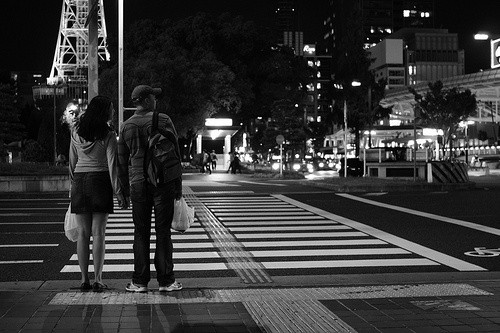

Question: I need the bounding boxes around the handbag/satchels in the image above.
[172,196,194,233]
[64,202,80,242]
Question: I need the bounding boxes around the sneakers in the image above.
[159,281,183,291]
[126,281,148,292]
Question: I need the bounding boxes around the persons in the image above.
[68,95,126,293]
[202,150,212,174]
[116,85,183,292]
[210,149,218,170]
[227,148,242,174]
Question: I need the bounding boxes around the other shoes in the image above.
[93,280,113,292]
[81,281,92,292]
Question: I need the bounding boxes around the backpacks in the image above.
[143,111,182,191]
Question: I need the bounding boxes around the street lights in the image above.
[473,30,491,42]
[351,81,362,87]
